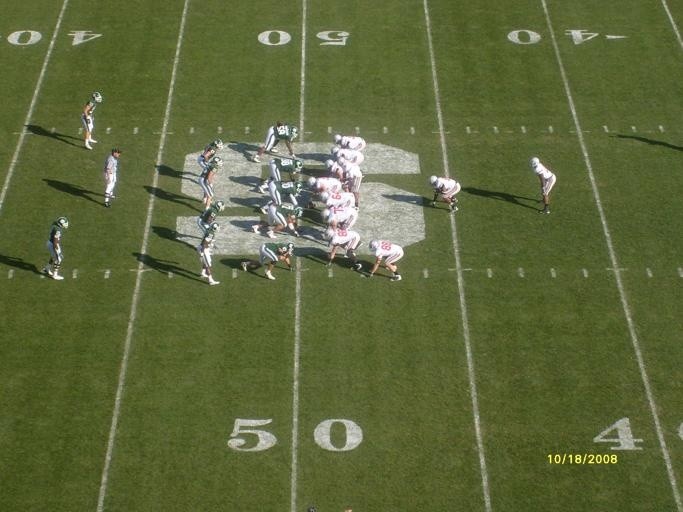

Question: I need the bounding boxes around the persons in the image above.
[368,240,404,281]
[241,121,367,281]
[104,148,123,208]
[530,157,556,214]
[81,91,103,150]
[45,216,70,282]
[430,175,461,212]
[197,137,225,287]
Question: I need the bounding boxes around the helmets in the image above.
[214,139,222,150]
[287,127,302,249]
[308,134,341,239]
[430,175,437,185]
[57,217,68,229]
[215,201,224,211]
[213,157,222,168]
[91,92,102,103]
[369,240,378,251]
[528,156,539,167]
[210,223,220,232]
